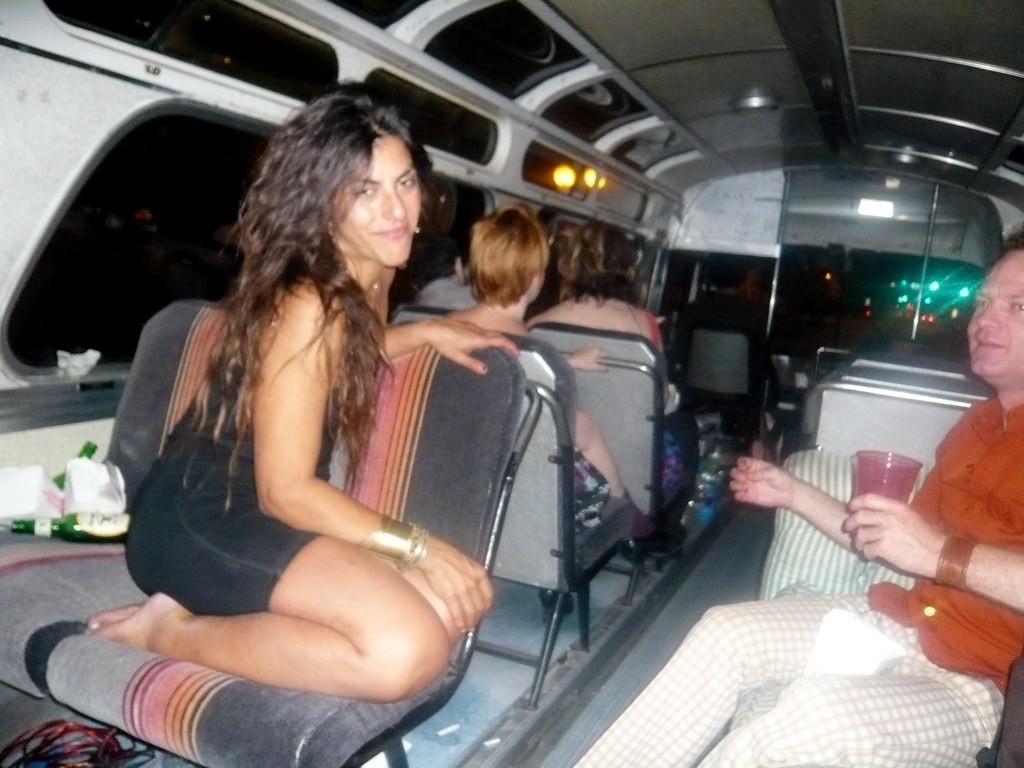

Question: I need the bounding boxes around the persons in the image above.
[714,264,783,451]
[448,202,654,549]
[85,89,520,703]
[413,254,478,309]
[526,216,684,503]
[572,227,1024,768]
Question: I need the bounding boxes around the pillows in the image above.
[758,449,935,601]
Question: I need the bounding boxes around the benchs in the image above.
[0,299,696,768]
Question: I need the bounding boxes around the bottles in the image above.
[13,512,129,543]
[54,441,96,490]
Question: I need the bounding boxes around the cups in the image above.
[855,450,924,506]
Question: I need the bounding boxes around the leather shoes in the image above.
[540,589,573,617]
[625,519,688,567]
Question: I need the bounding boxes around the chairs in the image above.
[685,326,769,451]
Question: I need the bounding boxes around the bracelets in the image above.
[936,536,976,590]
[370,517,429,568]
